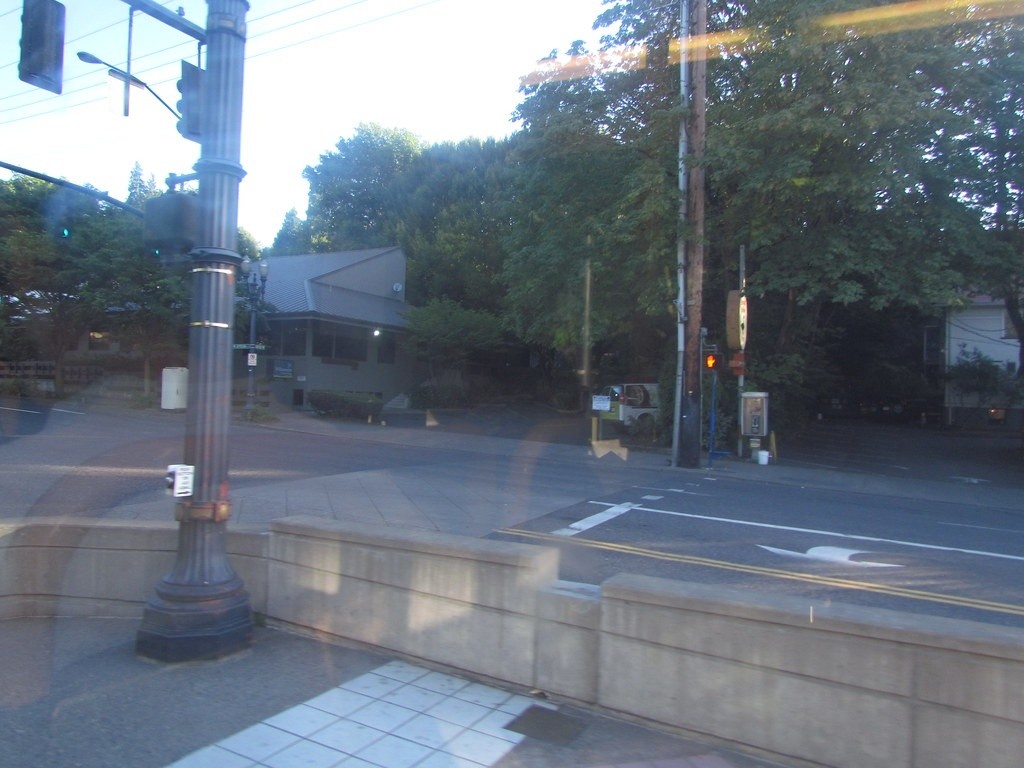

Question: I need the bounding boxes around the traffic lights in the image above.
[706,353,723,369]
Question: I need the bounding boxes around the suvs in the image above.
[598,381,665,437]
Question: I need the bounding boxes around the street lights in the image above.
[239,255,269,409]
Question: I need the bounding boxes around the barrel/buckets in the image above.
[758,450,769,465]
